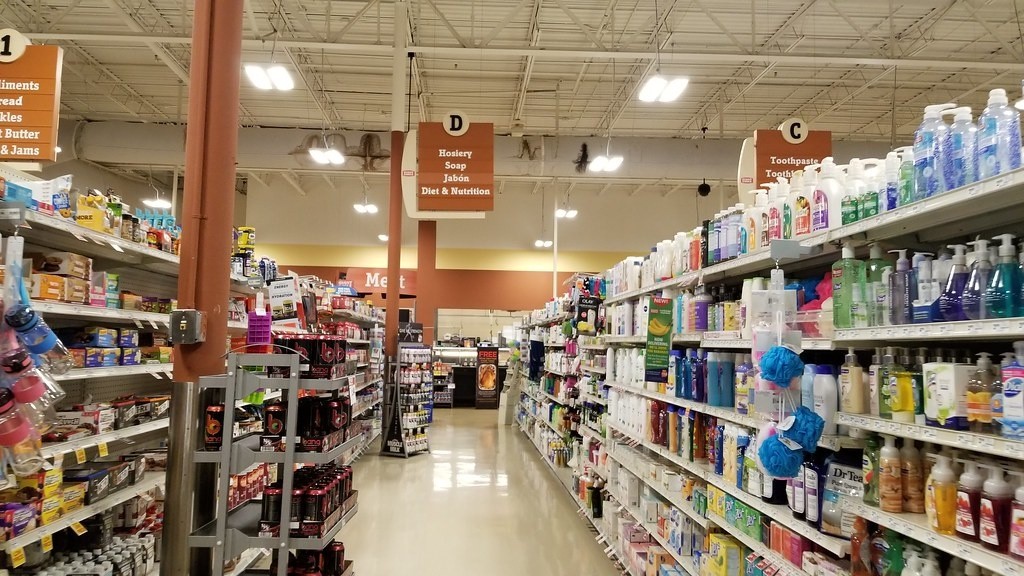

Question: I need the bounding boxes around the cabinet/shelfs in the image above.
[510,168,1024,575]
[0,202,390,576]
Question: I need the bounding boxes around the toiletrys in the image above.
[510,82,1024,576]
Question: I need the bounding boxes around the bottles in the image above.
[0,304,74,491]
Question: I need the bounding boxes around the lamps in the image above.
[243,35,293,92]
[352,186,378,214]
[307,123,345,166]
[639,40,688,103]
[556,195,577,220]
[588,138,623,172]
[534,229,553,248]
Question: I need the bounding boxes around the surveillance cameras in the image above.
[698,184,710,196]
